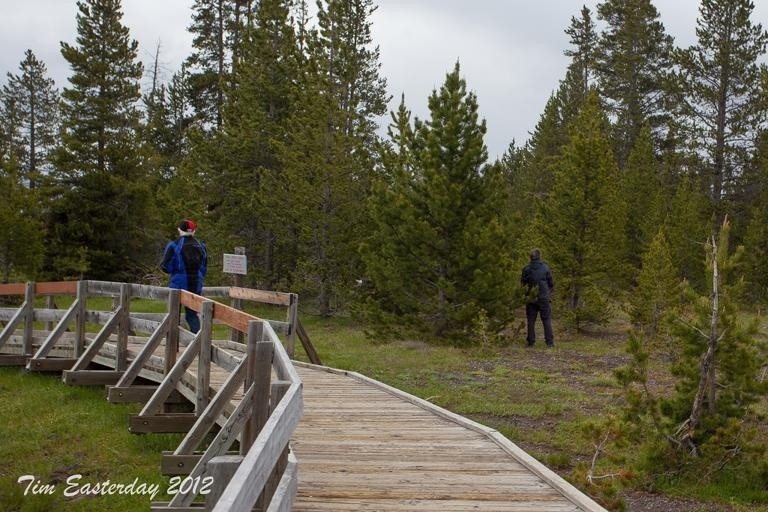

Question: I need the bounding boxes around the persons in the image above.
[158,219,208,335]
[520,248,556,348]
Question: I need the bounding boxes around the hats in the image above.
[180,220,195,232]
